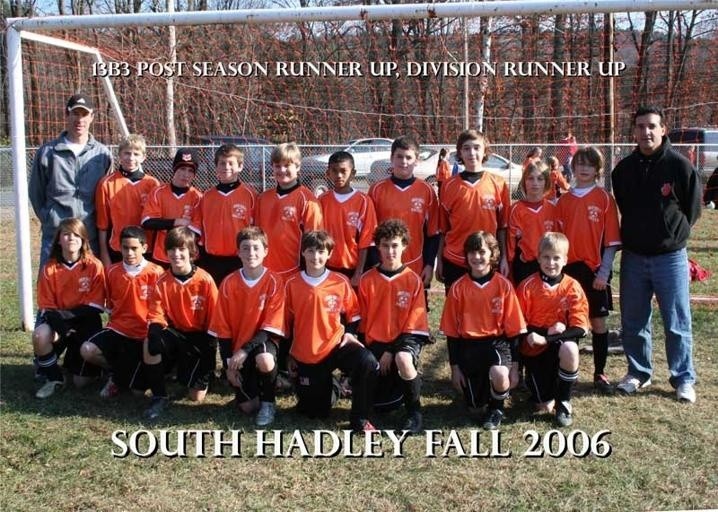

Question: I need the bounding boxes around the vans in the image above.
[666,127,718,183]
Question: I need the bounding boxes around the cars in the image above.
[366,144,523,197]
[300,138,396,177]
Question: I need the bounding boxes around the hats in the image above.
[66,94,94,114]
[173,148,199,178]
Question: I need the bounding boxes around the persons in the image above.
[437,148,450,199]
[79,226,164,398]
[208,227,290,426]
[33,219,105,400]
[523,147,543,169]
[286,231,382,431]
[141,149,204,270]
[518,232,589,426]
[684,145,695,164]
[567,136,577,181]
[704,155,718,207]
[506,161,557,284]
[28,95,113,283]
[543,156,571,197]
[256,142,323,277]
[435,129,511,295]
[613,106,702,404]
[614,132,636,161]
[558,146,623,393]
[439,231,528,429]
[202,144,258,287]
[358,220,430,428]
[556,129,573,183]
[318,151,378,286]
[368,136,439,311]
[143,227,218,401]
[96,134,160,267]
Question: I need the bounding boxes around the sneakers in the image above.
[533,398,555,418]
[572,378,580,394]
[351,413,384,436]
[676,382,696,403]
[555,400,573,427]
[143,394,168,423]
[402,411,423,434]
[616,374,652,394]
[98,367,107,384]
[219,367,230,386]
[208,371,219,384]
[99,377,120,400]
[274,372,293,395]
[593,374,615,396]
[339,370,352,399]
[36,370,72,399]
[288,361,306,388]
[255,402,276,426]
[189,388,207,402]
[165,369,179,384]
[483,409,505,430]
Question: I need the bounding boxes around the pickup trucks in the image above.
[142,135,330,198]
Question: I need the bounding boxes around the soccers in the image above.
[705,200,715,209]
